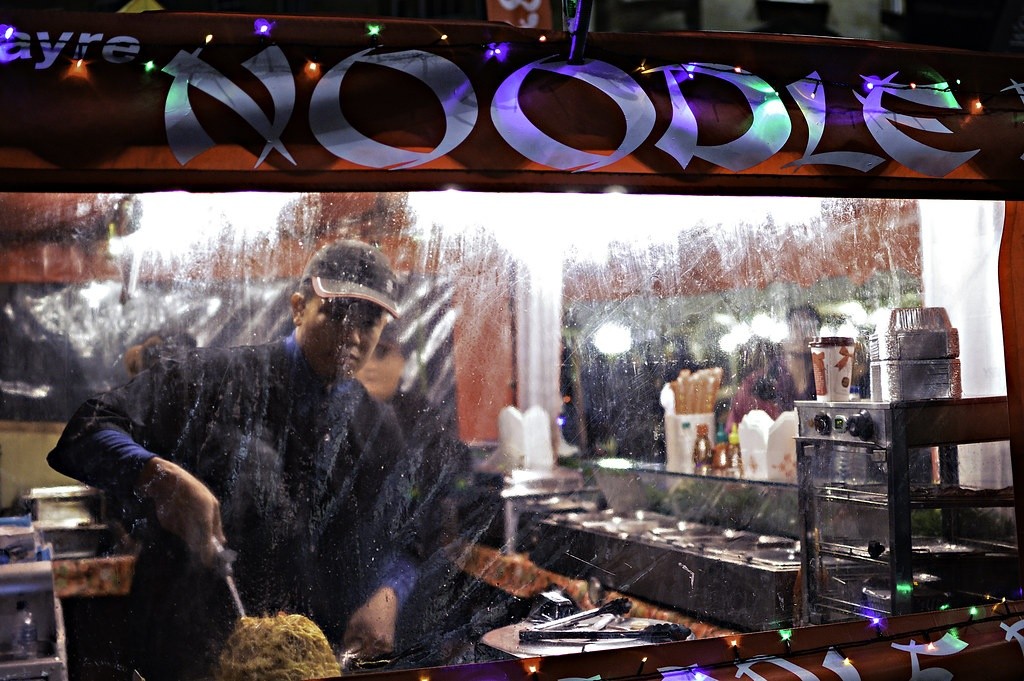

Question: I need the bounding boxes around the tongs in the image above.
[520,597,691,643]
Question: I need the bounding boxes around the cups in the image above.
[808,336,854,402]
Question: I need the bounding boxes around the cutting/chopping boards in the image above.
[481,616,694,657]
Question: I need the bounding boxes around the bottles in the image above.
[712,422,729,468]
[694,424,711,467]
[10,601,37,659]
[729,423,740,468]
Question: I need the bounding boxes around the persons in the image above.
[560,303,839,477]
[45,241,429,681]
[357,319,483,668]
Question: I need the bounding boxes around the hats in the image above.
[301,241,398,318]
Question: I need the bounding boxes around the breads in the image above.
[671,366,722,415]
